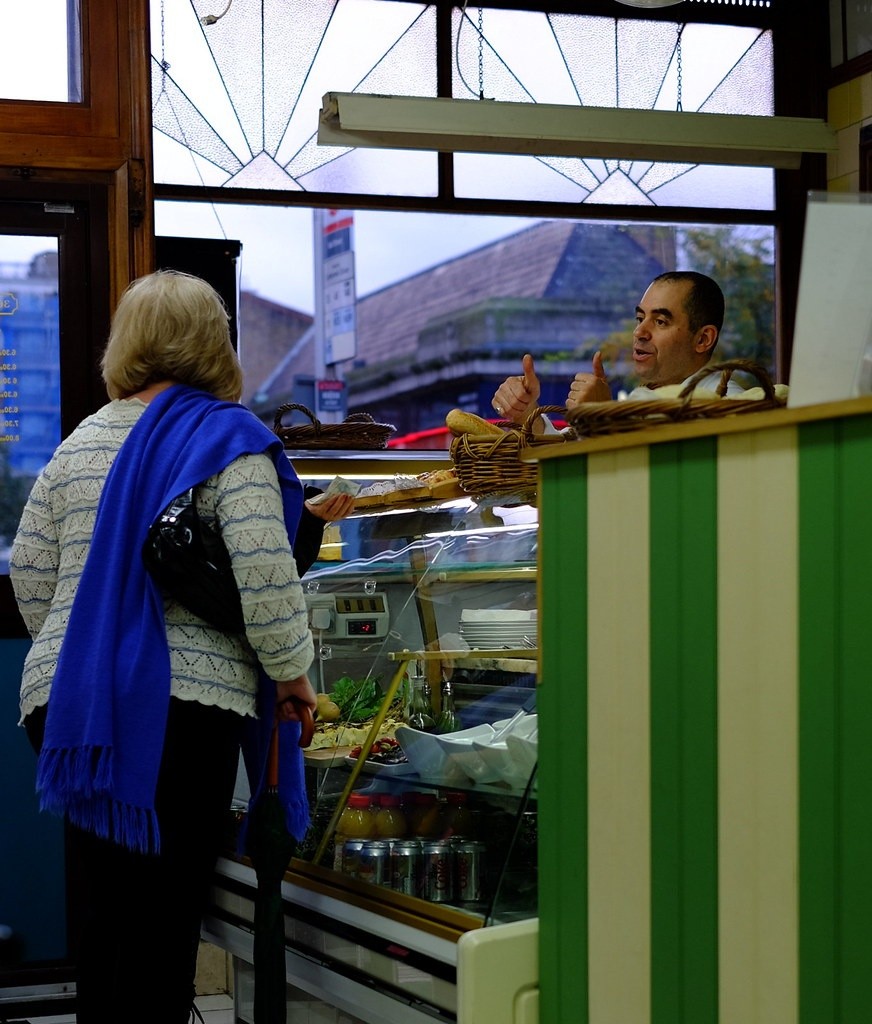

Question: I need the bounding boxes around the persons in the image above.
[491,271,745,426]
[10,271,354,1024]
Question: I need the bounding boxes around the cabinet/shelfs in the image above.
[200,485,539,1024]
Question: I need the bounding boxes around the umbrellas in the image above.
[246,697,315,1024]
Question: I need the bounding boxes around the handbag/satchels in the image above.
[144,482,247,640]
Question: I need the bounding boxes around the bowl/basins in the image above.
[394,715,538,791]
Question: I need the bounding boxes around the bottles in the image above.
[332,792,472,875]
[410,676,461,735]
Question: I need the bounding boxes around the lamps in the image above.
[317,0,839,171]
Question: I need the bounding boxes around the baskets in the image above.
[271,403,396,450]
[471,493,538,526]
[450,405,578,492]
[565,359,784,441]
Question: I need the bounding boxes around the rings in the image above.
[495,406,502,414]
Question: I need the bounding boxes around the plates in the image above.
[345,755,418,775]
[458,619,537,649]
